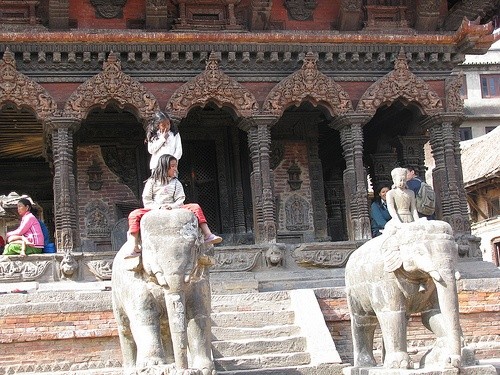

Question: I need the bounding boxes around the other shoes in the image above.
[204,234,222,245]
[124,251,141,259]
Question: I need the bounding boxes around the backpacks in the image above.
[413,178,435,215]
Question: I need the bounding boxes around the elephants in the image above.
[111,208,217,375]
[344,219,462,369]
